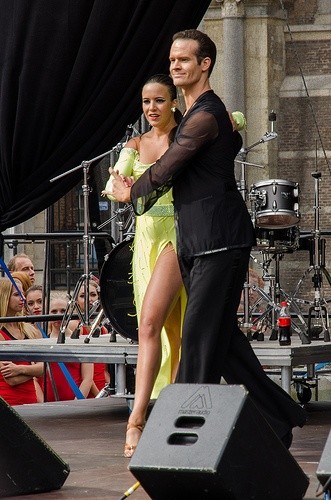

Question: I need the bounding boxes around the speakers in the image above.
[128,383,310,500]
[316,429,331,497]
[0,396,70,496]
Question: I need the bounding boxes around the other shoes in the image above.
[284,404,306,451]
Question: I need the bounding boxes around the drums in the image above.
[254,224,301,252]
[248,178,303,230]
[96,236,139,347]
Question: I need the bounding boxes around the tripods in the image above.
[49,141,122,344]
[239,178,331,345]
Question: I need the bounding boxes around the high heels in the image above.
[123,420,147,458]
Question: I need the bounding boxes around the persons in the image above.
[105,74,238,458]
[103,29,306,450]
[0,253,110,404]
[238,268,265,329]
